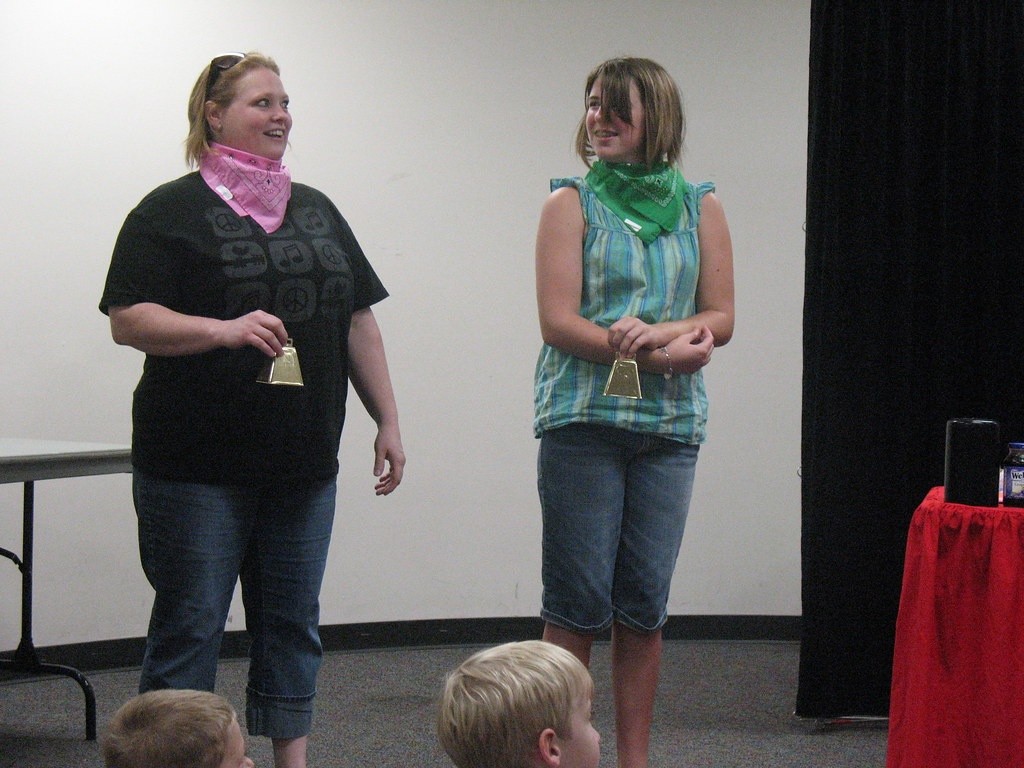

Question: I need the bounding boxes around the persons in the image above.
[98,52,407,768]
[103,689,255,768]
[436,640,602,768]
[531,56,736,768]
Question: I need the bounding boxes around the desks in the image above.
[0,437,131,741]
[887,485,1023,768]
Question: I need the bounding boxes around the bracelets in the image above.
[659,347,673,380]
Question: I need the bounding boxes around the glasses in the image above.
[205,52,245,104]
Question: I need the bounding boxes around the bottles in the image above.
[1001,442,1024,507]
